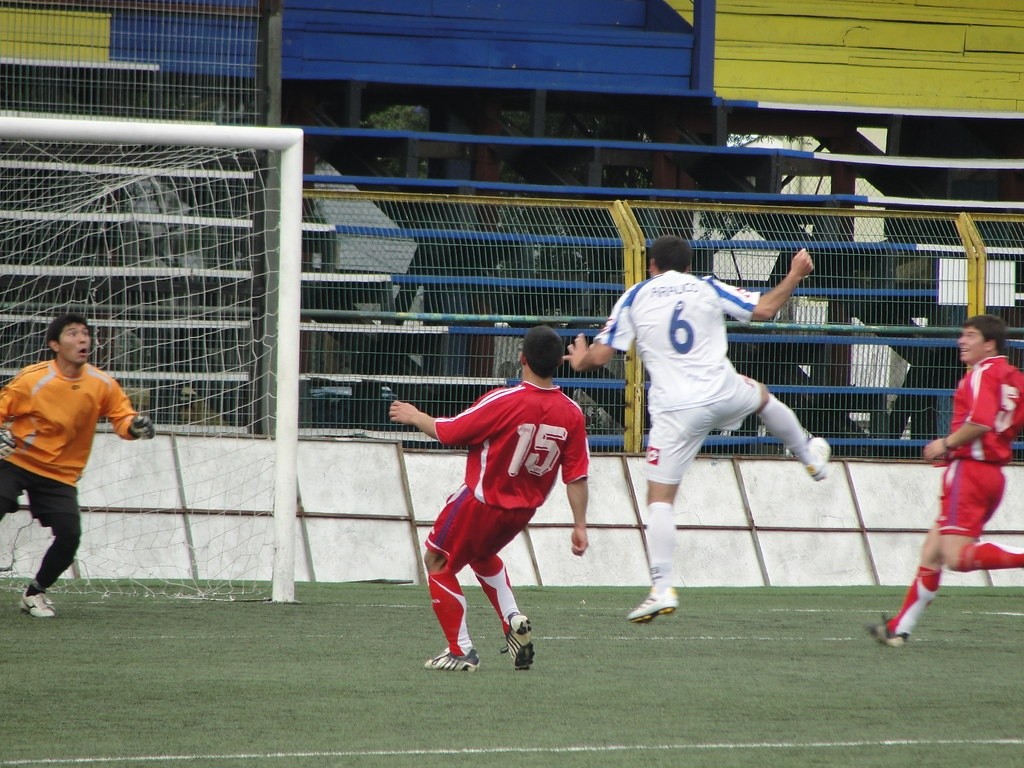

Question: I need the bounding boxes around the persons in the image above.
[387,327,590,672]
[862,314,1024,650]
[561,235,831,623]
[0,314,155,618]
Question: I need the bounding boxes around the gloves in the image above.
[0,428,15,460]
[130,416,155,439]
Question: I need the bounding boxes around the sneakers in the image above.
[867,621,909,647]
[805,437,831,479]
[425,648,480,672]
[627,587,678,623]
[21,588,56,617]
[505,612,534,670]
